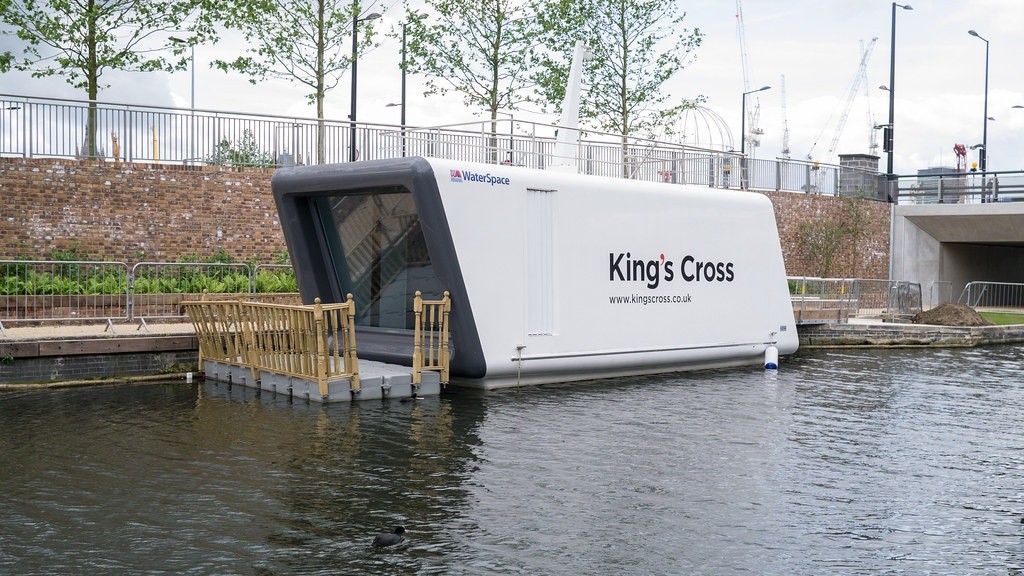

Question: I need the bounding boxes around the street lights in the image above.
[348,12,383,161]
[401,13,430,159]
[969,29,990,202]
[887,1,914,174]
[742,86,772,179]
[169,36,195,168]
